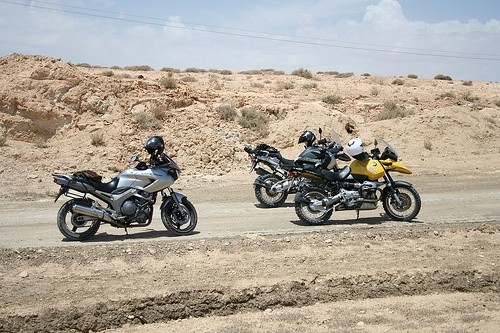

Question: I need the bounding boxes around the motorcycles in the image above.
[244,128,351,207]
[51,135,197,238]
[293,124,421,225]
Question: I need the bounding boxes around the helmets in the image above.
[146,135,164,154]
[298,130,316,148]
[346,138,363,156]
